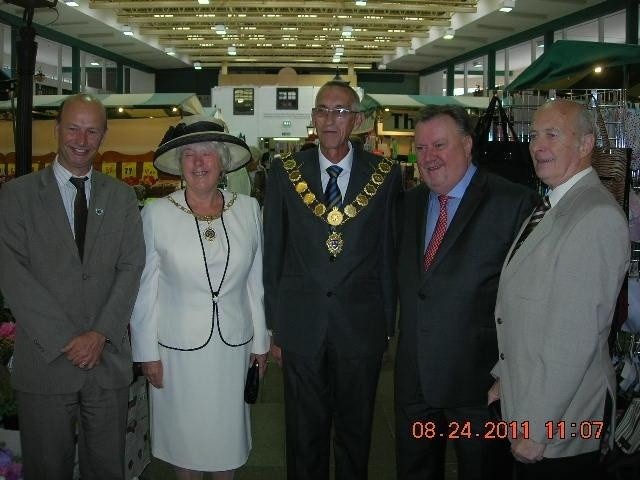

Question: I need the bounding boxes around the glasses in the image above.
[313,106,353,119]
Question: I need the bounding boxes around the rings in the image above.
[81,361,88,367]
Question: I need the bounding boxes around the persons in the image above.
[263,81,400,480]
[487,98,630,480]
[128,115,273,480]
[1,93,147,479]
[226,146,267,207]
[394,104,537,480]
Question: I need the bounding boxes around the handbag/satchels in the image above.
[244,359,261,404]
[591,147,631,211]
[479,140,537,190]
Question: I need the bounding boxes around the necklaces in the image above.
[280,151,394,259]
[164,190,238,243]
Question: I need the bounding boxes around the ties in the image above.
[326,165,344,214]
[68,174,88,263]
[507,194,551,264]
[423,195,449,269]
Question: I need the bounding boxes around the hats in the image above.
[152,116,252,177]
